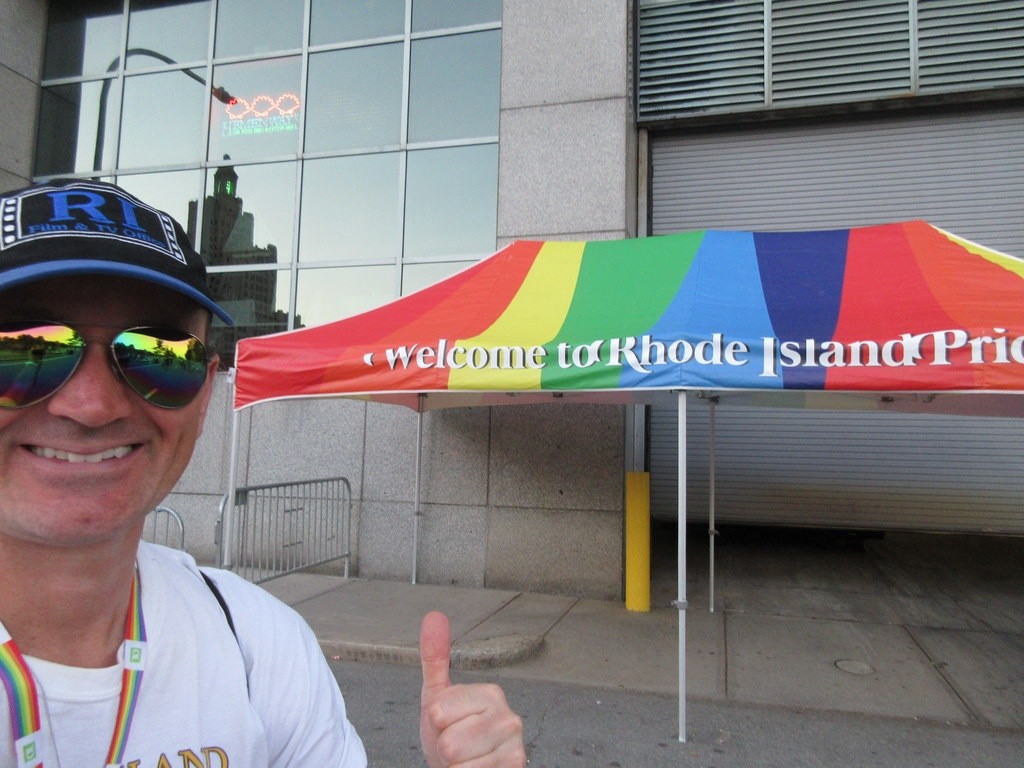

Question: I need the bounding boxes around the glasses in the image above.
[0,318,216,412]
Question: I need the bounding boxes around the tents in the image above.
[220,218,1024,745]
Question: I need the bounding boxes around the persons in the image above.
[0,179,527,768]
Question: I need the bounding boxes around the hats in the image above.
[0,178,237,329]
